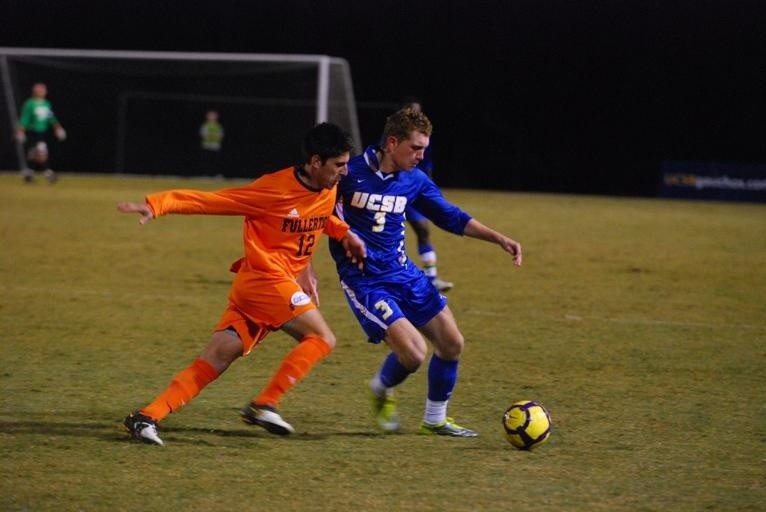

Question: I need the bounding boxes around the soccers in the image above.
[501,400,552,449]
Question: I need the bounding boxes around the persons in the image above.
[332,102,522,439]
[15,82,66,184]
[200,111,225,180]
[383,103,456,293]
[118,125,367,445]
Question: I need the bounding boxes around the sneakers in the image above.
[124,409,163,445]
[366,380,399,433]
[418,416,478,437]
[431,278,454,292]
[240,402,294,435]
